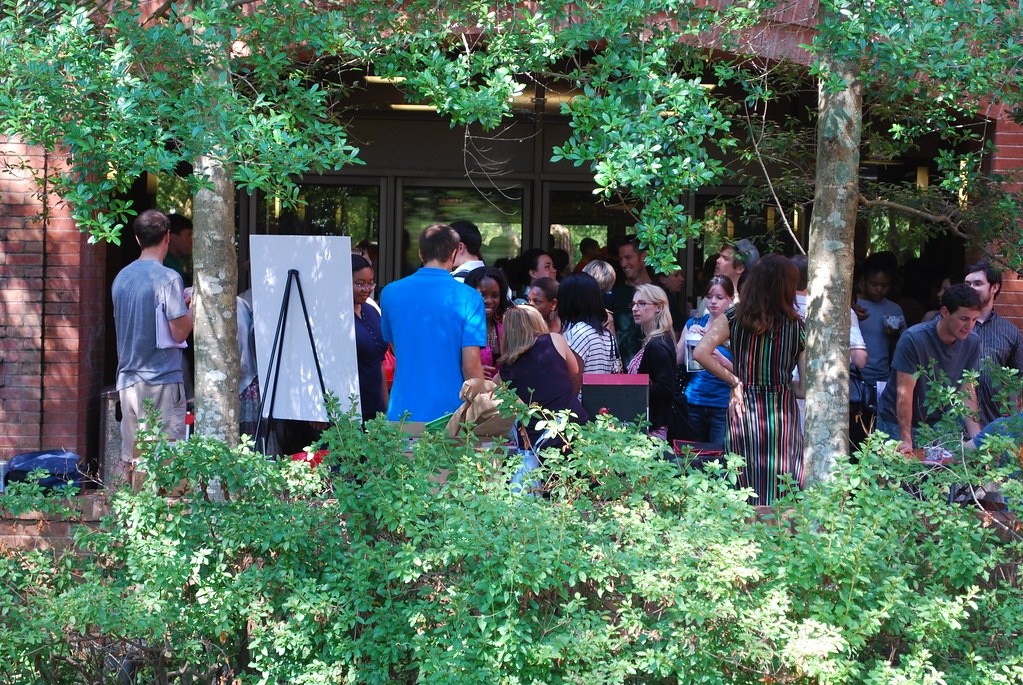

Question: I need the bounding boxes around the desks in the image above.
[907,446,982,511]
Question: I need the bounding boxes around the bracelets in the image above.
[729,376,741,390]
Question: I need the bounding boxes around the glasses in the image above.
[631,300,658,308]
[625,235,639,242]
[353,281,377,290]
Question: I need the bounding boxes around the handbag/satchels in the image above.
[849,362,877,433]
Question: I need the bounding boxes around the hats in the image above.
[735,238,760,276]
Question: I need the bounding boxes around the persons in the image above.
[573,238,601,271]
[381,226,487,423]
[627,283,679,445]
[527,277,565,335]
[693,252,808,507]
[110,208,192,488]
[162,214,194,404]
[698,238,760,313]
[547,248,571,279]
[448,219,485,283]
[235,295,280,459]
[464,266,512,381]
[555,271,620,404]
[678,274,736,445]
[582,260,617,340]
[487,304,600,498]
[506,248,557,306]
[310,252,389,451]
[612,235,686,374]
[789,248,1023,512]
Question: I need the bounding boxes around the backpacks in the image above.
[3,449,83,497]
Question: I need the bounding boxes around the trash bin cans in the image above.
[97,388,131,494]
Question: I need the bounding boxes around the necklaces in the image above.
[487,321,498,352]
